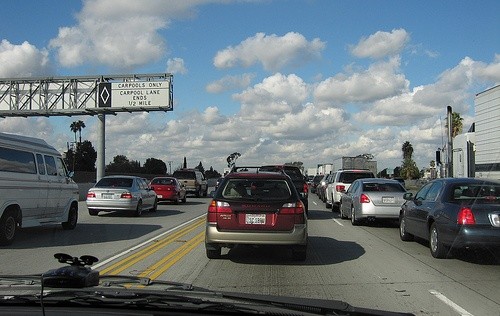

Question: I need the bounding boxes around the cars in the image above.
[338,178,409,226]
[399,177,500,259]
[205,166,309,260]
[86,176,159,217]
[305,173,337,202]
[148,177,187,204]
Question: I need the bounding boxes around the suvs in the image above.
[324,169,381,213]
[173,168,208,198]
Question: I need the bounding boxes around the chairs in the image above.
[475,186,491,197]
[462,189,472,196]
[230,184,247,196]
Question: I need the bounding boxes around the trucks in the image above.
[0,133,79,247]
